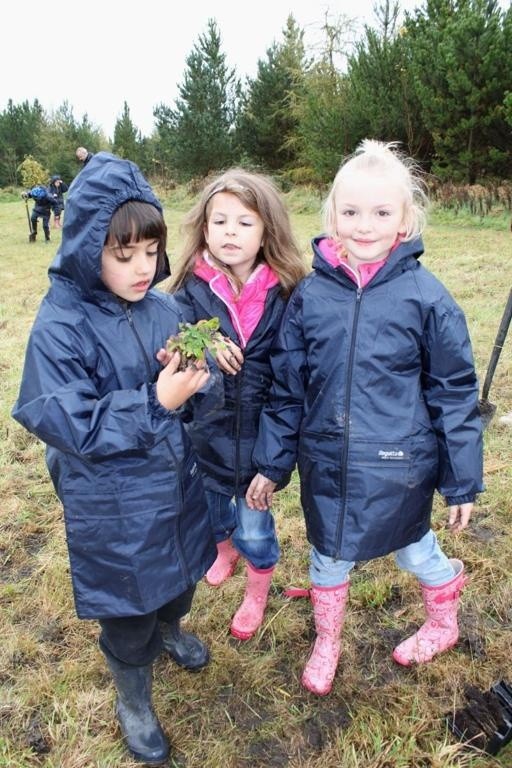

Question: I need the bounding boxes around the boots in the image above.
[30,220,39,235]
[228,557,276,641]
[392,556,466,667]
[97,630,171,765]
[154,615,211,674]
[44,230,52,244]
[204,535,243,587]
[281,580,352,696]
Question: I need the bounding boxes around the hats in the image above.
[29,186,46,200]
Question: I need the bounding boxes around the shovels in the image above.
[478,287,512,427]
[23,196,37,242]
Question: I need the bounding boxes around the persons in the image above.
[22,187,61,241]
[76,147,94,168]
[11,150,224,767]
[164,167,311,641]
[246,138,486,695]
[47,176,68,230]
